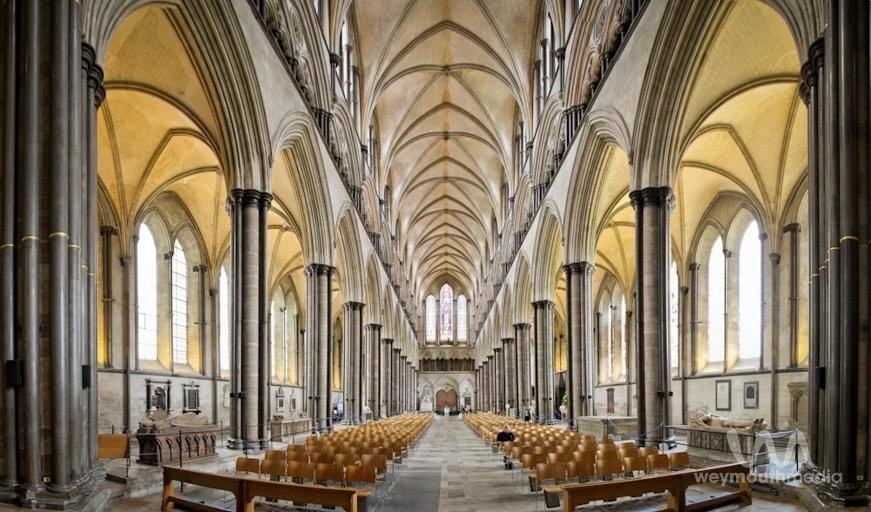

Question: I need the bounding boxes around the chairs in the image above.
[462,410,705,512]
[223,411,435,512]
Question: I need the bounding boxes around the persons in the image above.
[685,407,768,434]
[135,410,211,429]
[559,401,568,422]
[442,403,473,423]
[497,424,515,471]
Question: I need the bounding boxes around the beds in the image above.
[134,423,234,467]
[688,410,769,455]
